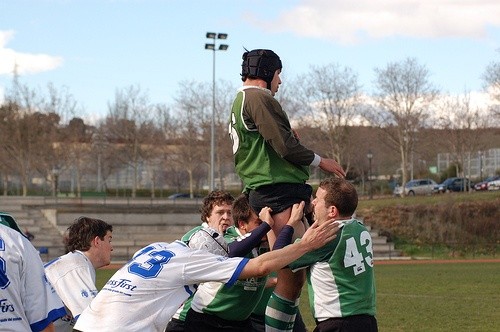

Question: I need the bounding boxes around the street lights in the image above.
[204,32,228,193]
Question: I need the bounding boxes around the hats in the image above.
[240,49,282,83]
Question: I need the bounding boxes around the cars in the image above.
[432,176,475,193]
[394,178,438,196]
[474,175,500,191]
[168,193,201,200]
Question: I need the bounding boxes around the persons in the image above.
[44,217,114,321]
[165,190,235,332]
[272,177,378,332]
[184,195,275,332]
[229,50,347,332]
[73,219,344,332]
[0,215,67,332]
